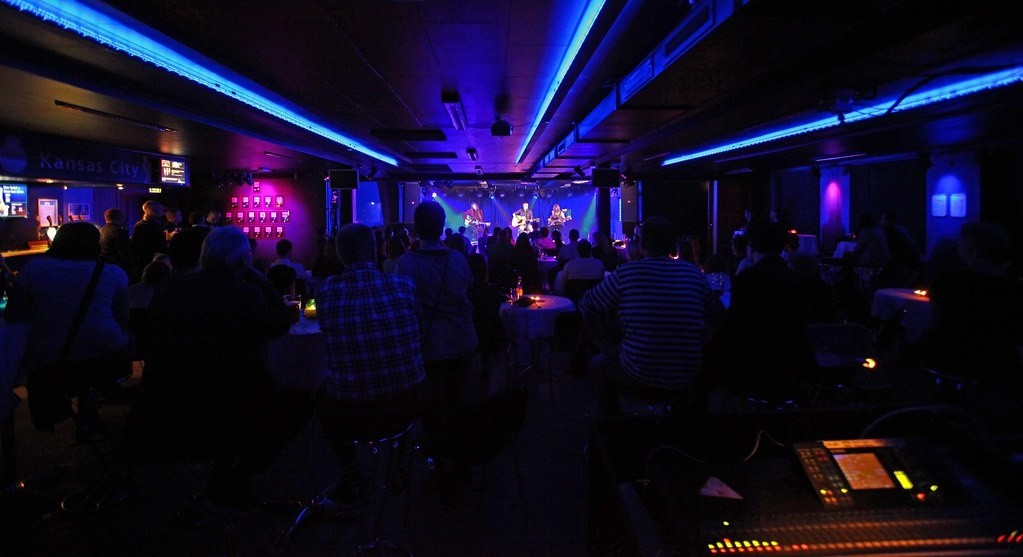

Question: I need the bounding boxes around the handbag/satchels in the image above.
[27,342,81,433]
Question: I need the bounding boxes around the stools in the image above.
[203,384,315,512]
[34,364,136,531]
[318,385,417,529]
[126,384,217,521]
[419,388,527,512]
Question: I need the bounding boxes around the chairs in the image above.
[870,309,908,370]
[564,278,602,304]
[808,323,876,374]
[536,312,581,399]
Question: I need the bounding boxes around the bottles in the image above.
[515,276,523,298]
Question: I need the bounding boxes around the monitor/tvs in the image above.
[158,156,188,187]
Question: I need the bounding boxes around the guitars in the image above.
[511,214,541,228]
[464,217,492,228]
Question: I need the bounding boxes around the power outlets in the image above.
[466,148,479,162]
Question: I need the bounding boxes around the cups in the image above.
[284,294,302,324]
[510,289,519,305]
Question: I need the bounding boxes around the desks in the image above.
[831,241,857,259]
[869,289,937,358]
[781,234,819,259]
[262,310,330,397]
[535,256,558,290]
[498,294,576,385]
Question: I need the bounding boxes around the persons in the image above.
[0,147,1023,513]
[462,202,483,236]
[548,204,566,231]
[512,202,534,232]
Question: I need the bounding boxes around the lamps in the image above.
[620,172,636,187]
[574,167,585,178]
[244,177,254,186]
[365,169,378,180]
[440,92,468,130]
[475,167,483,175]
